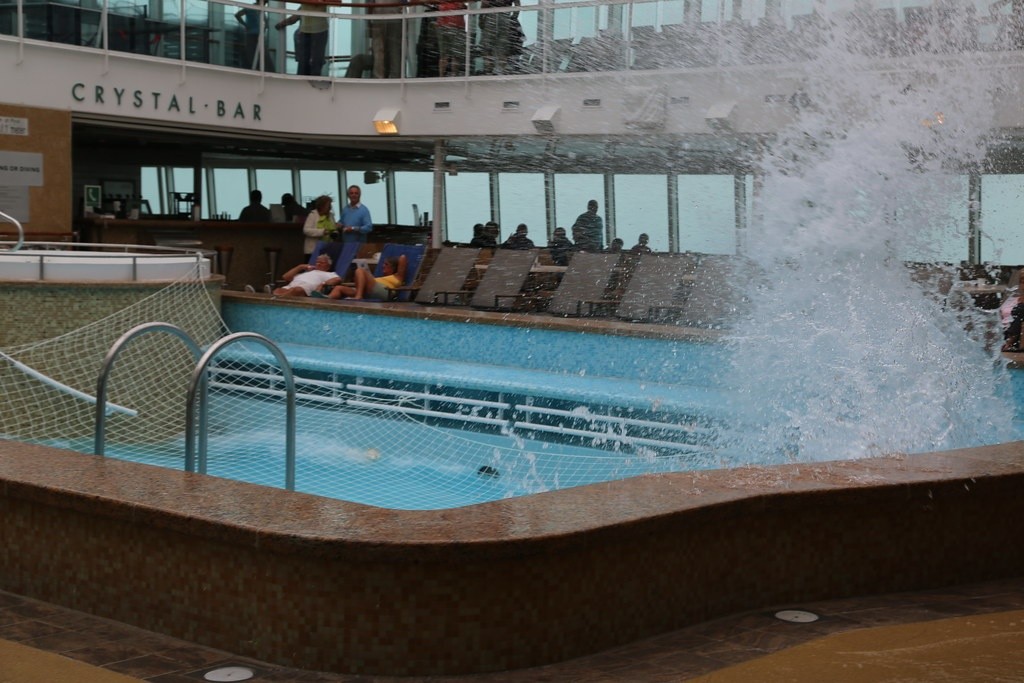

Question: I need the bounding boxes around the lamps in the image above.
[373,108,403,135]
[705,102,738,130]
[529,108,562,134]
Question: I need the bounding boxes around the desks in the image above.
[950,286,1006,311]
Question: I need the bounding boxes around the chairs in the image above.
[515,5,975,74]
[273,241,756,328]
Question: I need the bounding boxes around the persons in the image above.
[233,0,277,73]
[310,253,407,301]
[274,0,330,76]
[569,199,605,249]
[470,221,499,245]
[605,238,624,253]
[280,192,308,221]
[334,184,374,234]
[244,254,344,296]
[630,233,652,252]
[303,194,338,250]
[413,0,524,75]
[502,223,535,247]
[545,227,574,267]
[238,190,272,223]
[365,0,404,77]
